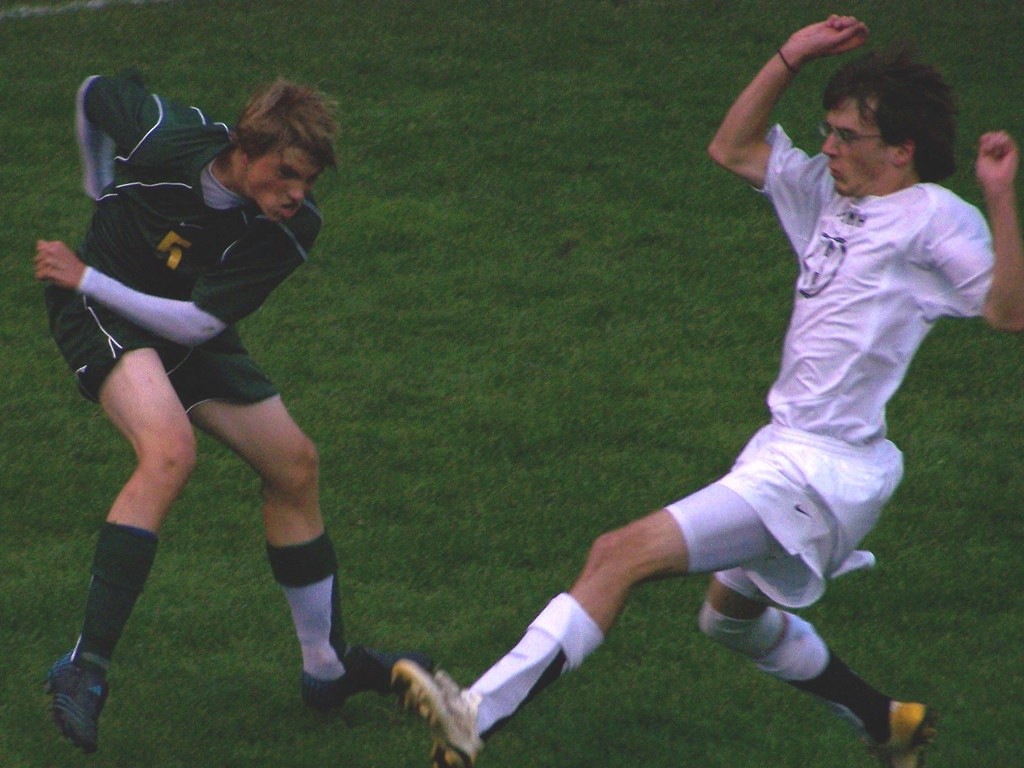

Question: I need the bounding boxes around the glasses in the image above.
[818,119,884,144]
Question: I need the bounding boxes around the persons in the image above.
[387,13,1024,768]
[28,72,435,757]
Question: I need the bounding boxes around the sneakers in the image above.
[301,644,434,713]
[390,655,486,768]
[862,699,939,768]
[45,649,109,754]
